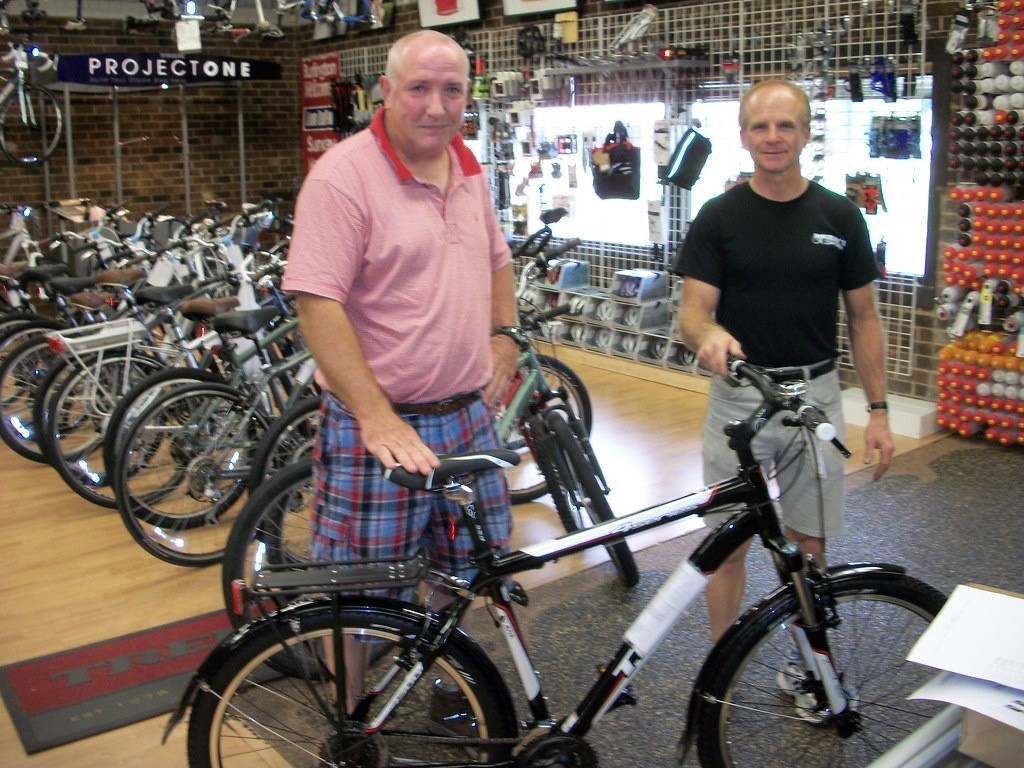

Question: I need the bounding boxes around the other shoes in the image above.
[430,684,484,745]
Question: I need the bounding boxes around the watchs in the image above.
[865,402,887,411]
[489,325,530,353]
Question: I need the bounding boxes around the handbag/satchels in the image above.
[588,129,641,200]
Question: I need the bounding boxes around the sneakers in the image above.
[777,659,830,724]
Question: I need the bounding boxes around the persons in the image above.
[279,30,521,762]
[675,78,895,723]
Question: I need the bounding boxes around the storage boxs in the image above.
[517,257,715,377]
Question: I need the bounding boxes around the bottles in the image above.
[936,0,1024,447]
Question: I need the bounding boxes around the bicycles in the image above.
[0,8,63,170]
[0,192,640,589]
[162,350,951,768]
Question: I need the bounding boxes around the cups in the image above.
[844,67,864,104]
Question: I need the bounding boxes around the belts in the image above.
[390,389,480,416]
[728,360,834,384]
